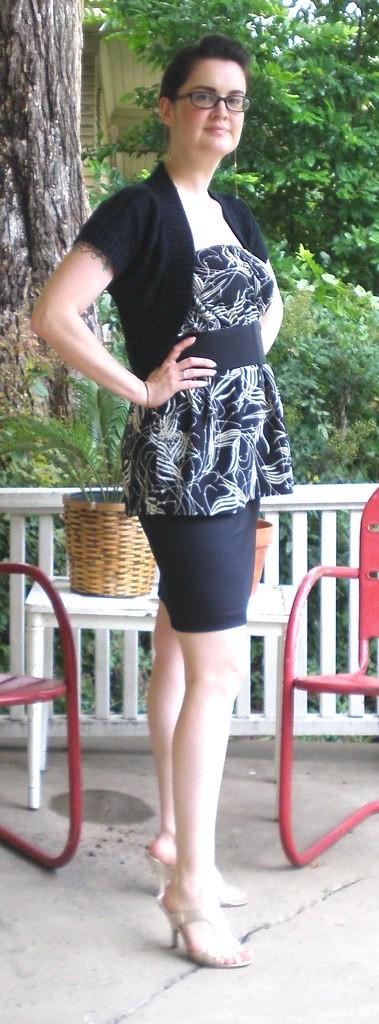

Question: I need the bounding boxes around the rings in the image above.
[183,370,188,379]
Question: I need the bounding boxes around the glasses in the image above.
[172,90,253,113]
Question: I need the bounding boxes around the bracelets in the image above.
[144,382,149,405]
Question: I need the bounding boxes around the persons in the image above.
[29,33,295,969]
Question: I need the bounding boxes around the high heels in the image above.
[146,831,248,909]
[156,890,253,968]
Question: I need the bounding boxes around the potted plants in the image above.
[0,338,157,598]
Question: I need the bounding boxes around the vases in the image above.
[248,519,273,596]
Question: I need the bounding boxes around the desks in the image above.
[23,574,299,821]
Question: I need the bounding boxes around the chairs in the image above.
[279,486,379,865]
[0,562,83,871]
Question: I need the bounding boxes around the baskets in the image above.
[62,490,155,599]
